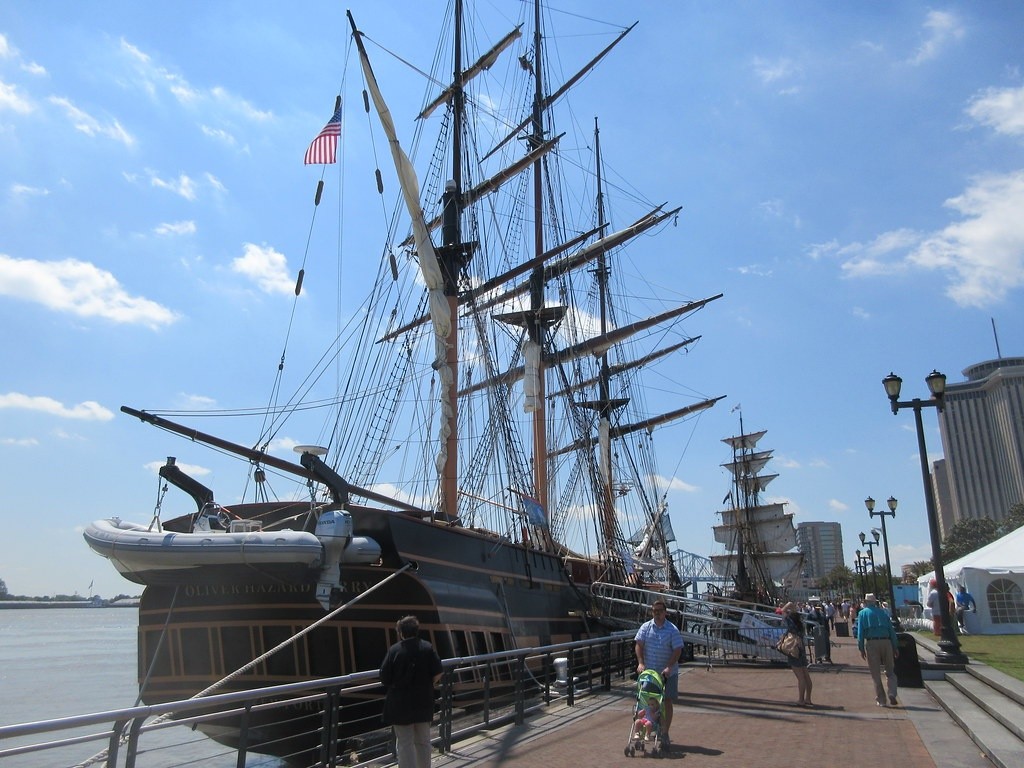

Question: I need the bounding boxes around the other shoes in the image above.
[645,734,649,741]
[825,657,831,661]
[876,701,886,707]
[817,658,822,664]
[634,732,640,740]
[661,735,669,746]
[888,693,898,705]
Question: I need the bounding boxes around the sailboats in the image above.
[84,2,730,764]
[690,409,809,641]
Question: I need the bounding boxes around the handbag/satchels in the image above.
[776,625,802,659]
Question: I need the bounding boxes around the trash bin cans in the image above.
[887,632,924,690]
[834,621,849,637]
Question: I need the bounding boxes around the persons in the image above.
[631,697,663,742]
[903,599,923,618]
[303,96,343,167]
[767,592,900,665]
[784,612,814,707]
[378,614,444,768]
[857,593,900,707]
[633,600,685,753]
[926,578,976,636]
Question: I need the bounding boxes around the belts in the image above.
[867,637,890,641]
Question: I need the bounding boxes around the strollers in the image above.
[624,670,672,760]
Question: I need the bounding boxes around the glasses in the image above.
[654,609,665,612]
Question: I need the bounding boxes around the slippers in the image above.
[790,701,813,707]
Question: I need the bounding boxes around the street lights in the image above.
[865,494,905,633]
[859,531,880,608]
[854,559,867,599]
[856,548,872,593]
[882,369,970,665]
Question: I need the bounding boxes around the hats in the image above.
[864,593,876,601]
[814,603,821,608]
